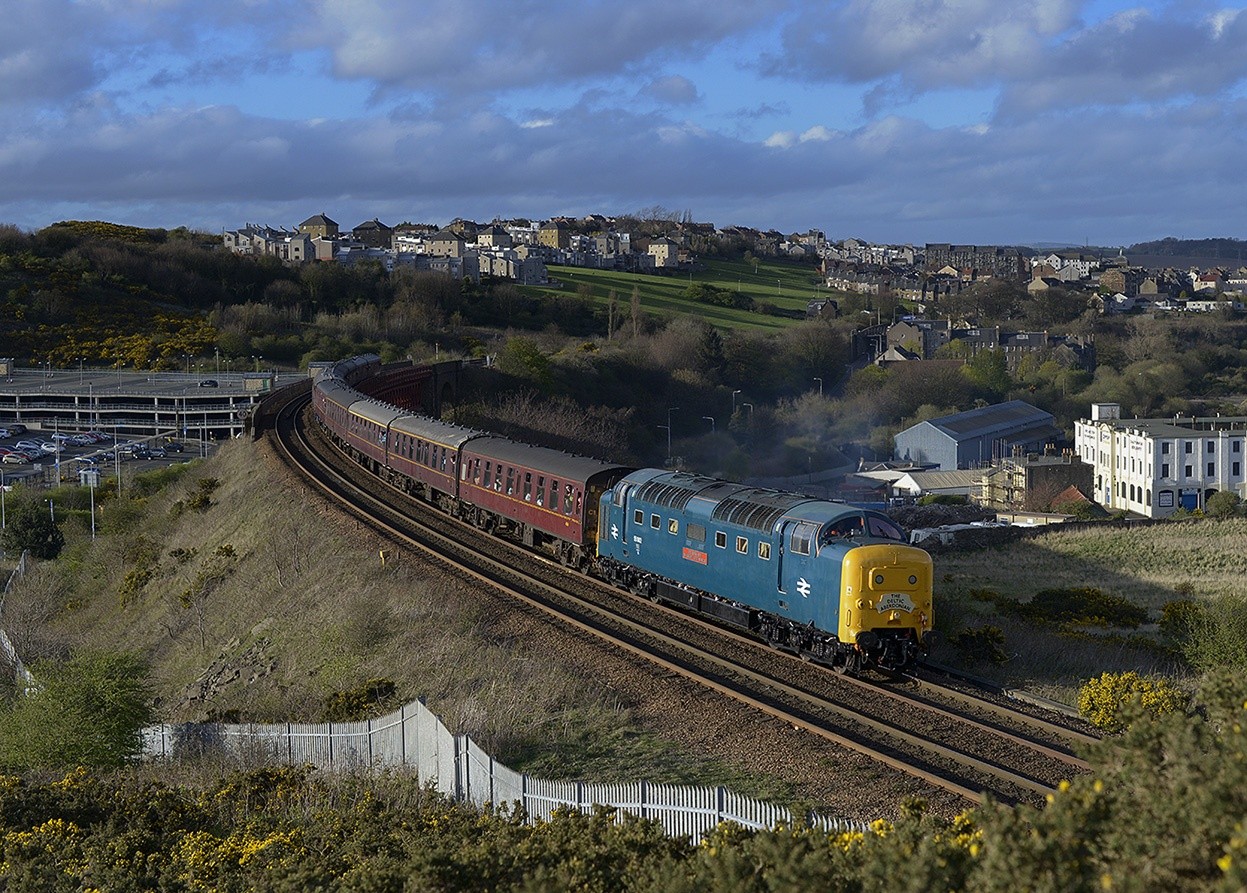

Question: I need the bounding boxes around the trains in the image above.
[311,350,938,678]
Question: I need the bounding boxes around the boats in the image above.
[1,422,191,493]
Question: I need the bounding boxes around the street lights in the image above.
[3,346,267,392]
